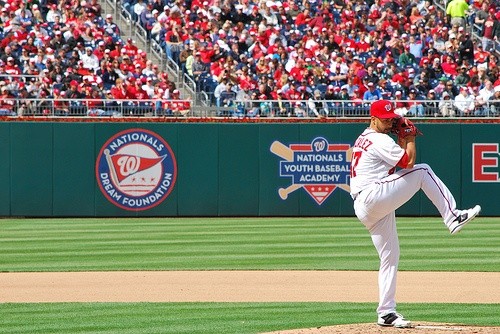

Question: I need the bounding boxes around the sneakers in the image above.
[449,204,481,234]
[378,313,414,329]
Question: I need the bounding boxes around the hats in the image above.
[370,101,400,119]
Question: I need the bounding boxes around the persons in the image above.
[0,0,192,117]
[350,101,481,326]
[113,0,500,117]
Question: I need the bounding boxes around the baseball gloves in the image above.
[390,115,424,139]
